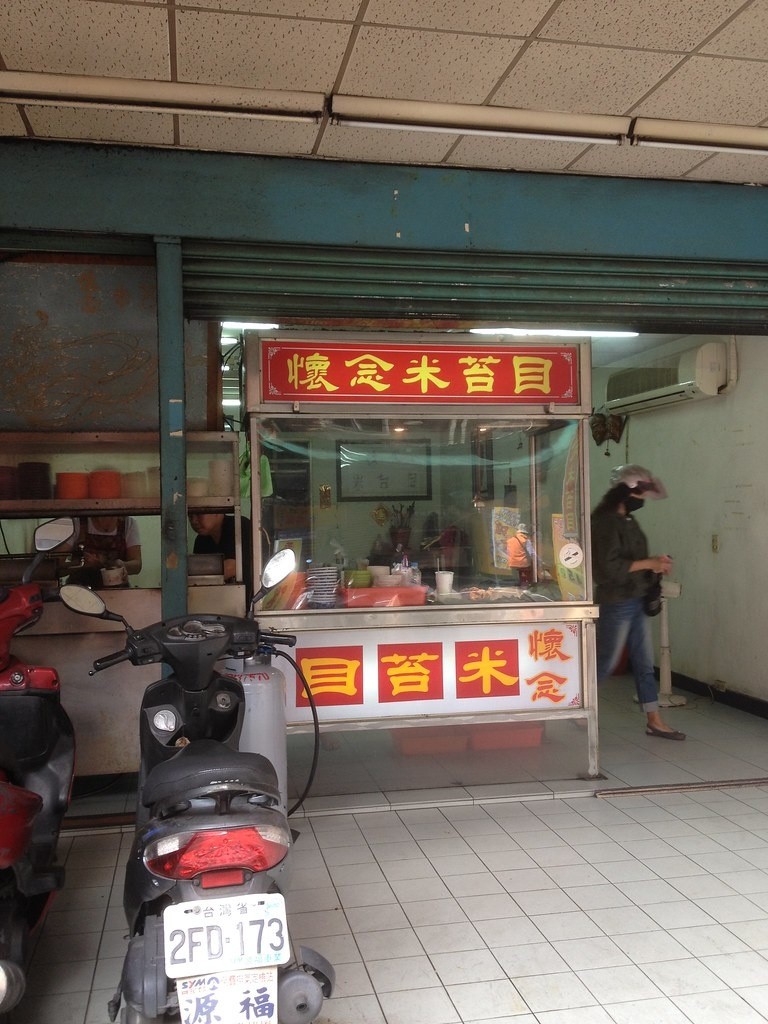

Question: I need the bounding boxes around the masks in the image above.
[623,496,644,513]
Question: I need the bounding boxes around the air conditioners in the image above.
[605,334,737,417]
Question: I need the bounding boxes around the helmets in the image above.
[609,463,668,500]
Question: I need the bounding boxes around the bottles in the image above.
[401,555,409,572]
[410,562,421,585]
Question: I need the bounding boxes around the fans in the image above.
[633,573,688,707]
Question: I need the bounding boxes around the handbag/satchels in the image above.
[644,570,663,616]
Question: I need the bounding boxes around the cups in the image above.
[435,571,454,603]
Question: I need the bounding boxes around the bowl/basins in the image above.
[146,467,160,497]
[56,473,88,499]
[0,466,18,499]
[208,461,232,496]
[89,471,120,499]
[123,472,146,498]
[344,565,401,588]
[18,462,50,498]
[101,567,124,585]
[307,567,338,608]
[188,479,209,497]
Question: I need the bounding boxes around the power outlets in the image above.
[713,680,726,692]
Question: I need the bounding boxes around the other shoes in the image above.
[646,723,685,740]
[571,719,600,732]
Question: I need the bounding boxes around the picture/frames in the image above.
[335,438,433,502]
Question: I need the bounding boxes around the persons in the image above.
[187,511,272,585]
[53,516,142,588]
[506,523,536,588]
[591,464,688,743]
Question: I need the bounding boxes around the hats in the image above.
[517,524,528,533]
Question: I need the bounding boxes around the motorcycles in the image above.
[0,514,80,1024]
[57,549,335,1024]
